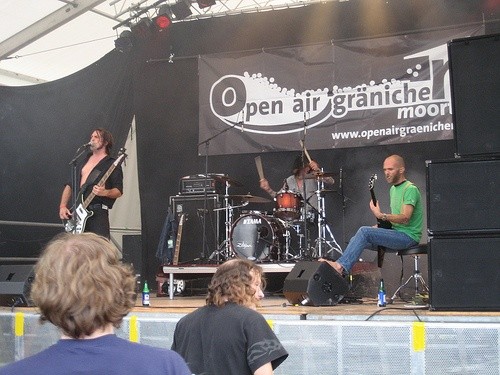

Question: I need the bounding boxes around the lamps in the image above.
[114,0,193,54]
[196,0,216,9]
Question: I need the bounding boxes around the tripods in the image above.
[279,177,344,262]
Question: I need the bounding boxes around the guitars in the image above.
[368,173,392,268]
[62,151,127,235]
[173,213,190,265]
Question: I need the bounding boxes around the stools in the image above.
[397,245,429,309]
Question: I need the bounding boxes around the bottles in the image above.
[376,279,386,307]
[141,280,149,307]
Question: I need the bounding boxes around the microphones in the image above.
[304,112,306,135]
[241,110,244,132]
[197,209,208,214]
[83,142,92,147]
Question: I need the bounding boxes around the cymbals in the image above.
[299,171,338,180]
[219,194,272,204]
[210,175,243,188]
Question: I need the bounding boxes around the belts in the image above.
[86,203,109,211]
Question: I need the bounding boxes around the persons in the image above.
[260,154,335,211]
[318,155,423,276]
[60,128,123,240]
[172,259,289,375]
[0,232,192,375]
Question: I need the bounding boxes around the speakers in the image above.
[283,261,350,308]
[425,32,500,313]
[0,265,36,307]
[169,195,227,264]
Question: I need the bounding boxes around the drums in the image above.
[275,191,302,220]
[230,214,291,262]
